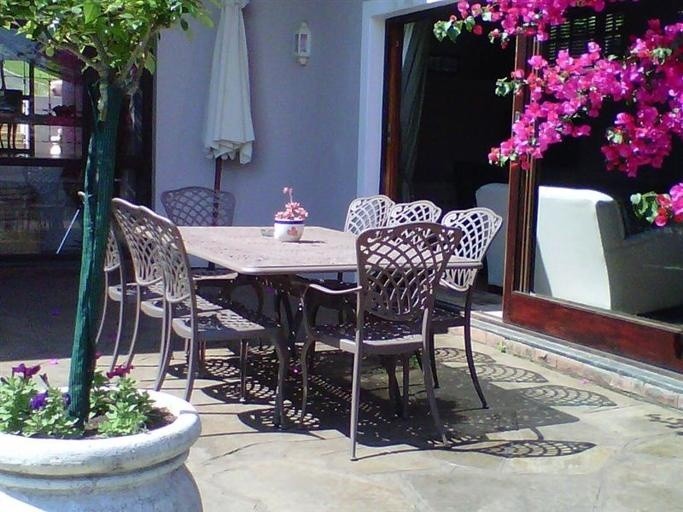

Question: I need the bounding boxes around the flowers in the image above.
[0,352,169,441]
[272,186,309,221]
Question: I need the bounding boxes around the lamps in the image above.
[290,19,312,68]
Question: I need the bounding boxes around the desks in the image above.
[173,223,485,437]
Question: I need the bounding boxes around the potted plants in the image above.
[0,0,209,511]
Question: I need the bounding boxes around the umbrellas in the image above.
[182,1,256,284]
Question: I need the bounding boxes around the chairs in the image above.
[111,197,220,383]
[158,185,239,306]
[135,205,265,403]
[316,199,444,355]
[294,222,464,461]
[296,193,395,351]
[375,201,502,412]
[76,191,161,372]
[0,160,73,236]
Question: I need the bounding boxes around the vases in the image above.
[274,219,304,242]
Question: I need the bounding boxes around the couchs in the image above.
[474,180,683,317]
